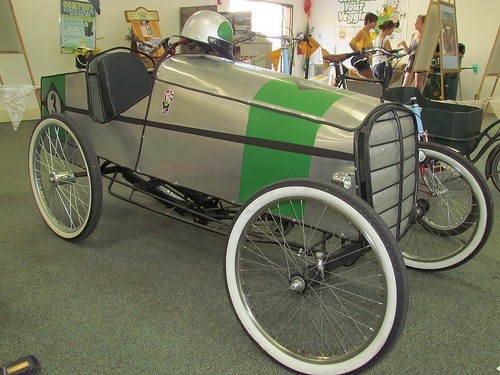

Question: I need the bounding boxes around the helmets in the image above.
[173,10,236,61]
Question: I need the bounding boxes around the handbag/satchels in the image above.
[378,66,393,84]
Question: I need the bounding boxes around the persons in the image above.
[373,18,396,85]
[349,13,379,78]
[398,15,426,95]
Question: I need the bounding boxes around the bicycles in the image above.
[322,48,410,99]
